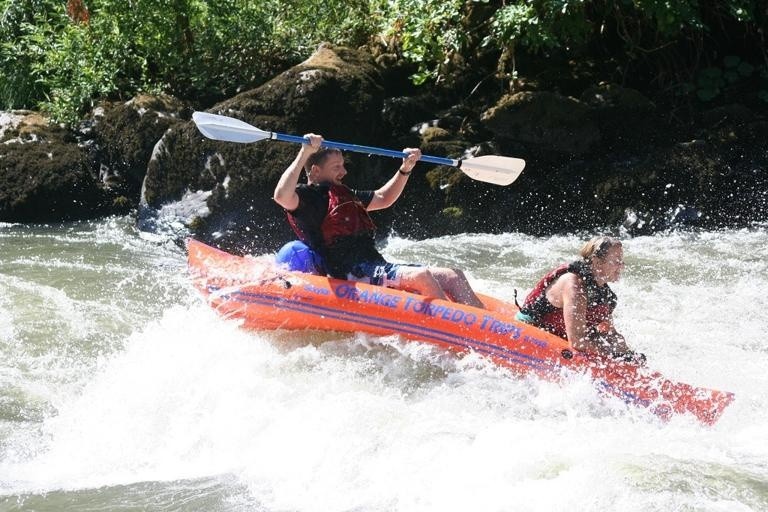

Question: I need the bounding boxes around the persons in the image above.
[272,130,488,310]
[515,234,647,369]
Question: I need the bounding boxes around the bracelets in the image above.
[398,168,413,176]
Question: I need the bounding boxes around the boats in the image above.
[180,235,735,430]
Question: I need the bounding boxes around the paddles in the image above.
[192,110,526,186]
